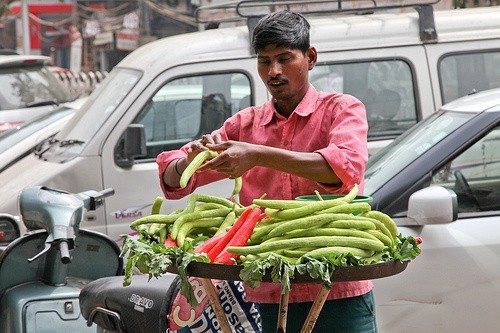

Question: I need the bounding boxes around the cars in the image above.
[0,55,250,174]
[364,88,500,333]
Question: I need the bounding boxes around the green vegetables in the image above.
[119,232,421,307]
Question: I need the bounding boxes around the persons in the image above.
[156,11,379,332]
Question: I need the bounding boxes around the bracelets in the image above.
[175,157,184,178]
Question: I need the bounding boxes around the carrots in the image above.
[194,207,267,266]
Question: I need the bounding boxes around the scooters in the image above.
[0,185,180,332]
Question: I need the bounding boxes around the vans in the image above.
[0,5,500,274]
